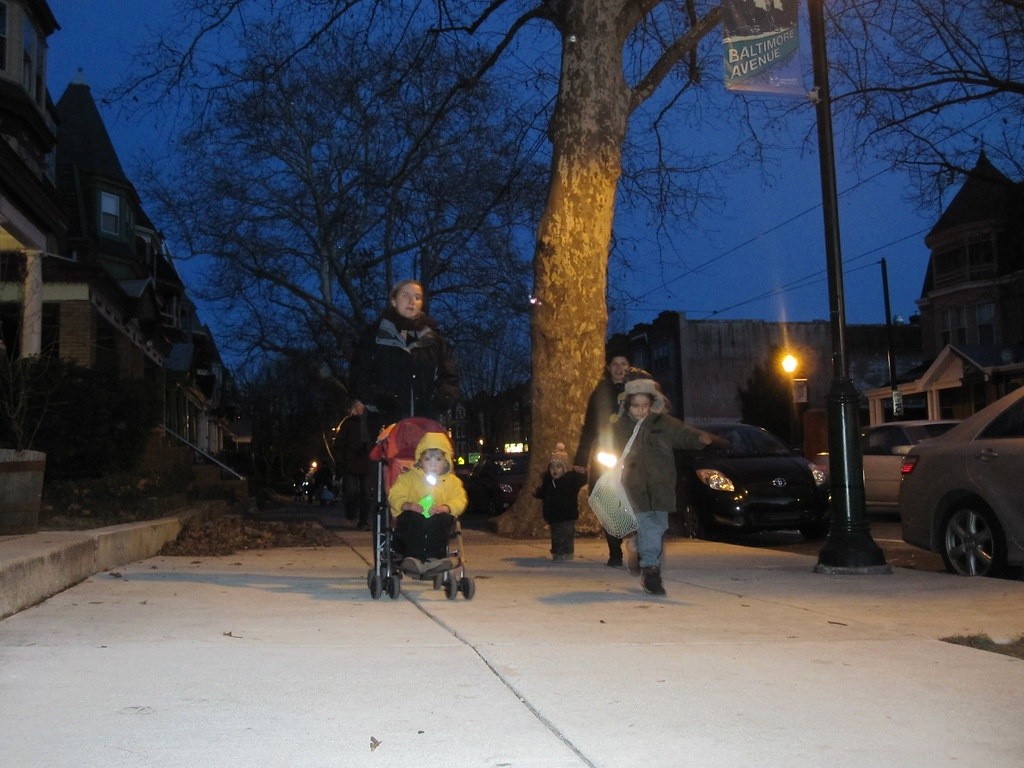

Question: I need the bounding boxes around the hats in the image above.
[548,443,569,488]
[618,367,665,418]
[605,333,635,369]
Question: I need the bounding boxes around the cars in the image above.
[818,418,966,516]
[474,453,531,513]
[671,424,831,542]
[898,387,1024,578]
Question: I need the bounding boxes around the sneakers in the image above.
[625,540,643,574]
[640,566,667,598]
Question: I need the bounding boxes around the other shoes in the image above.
[553,553,562,563]
[422,557,453,579]
[401,557,425,580]
[606,556,622,567]
[563,552,574,561]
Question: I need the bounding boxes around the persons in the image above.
[610,367,713,594]
[387,433,467,580]
[532,443,587,562]
[347,281,461,571]
[471,453,495,517]
[332,416,377,531]
[573,348,660,567]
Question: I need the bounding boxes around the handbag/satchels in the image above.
[588,469,638,540]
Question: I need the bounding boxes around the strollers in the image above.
[367,417,475,599]
